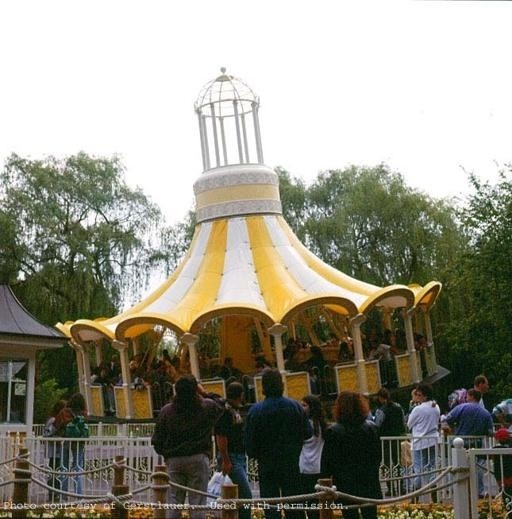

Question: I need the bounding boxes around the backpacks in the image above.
[66,414,88,439]
[271,441,304,484]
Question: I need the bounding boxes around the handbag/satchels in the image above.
[208,471,232,501]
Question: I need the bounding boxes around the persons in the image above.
[87,333,431,416]
[43,393,89,503]
[151,368,512,519]
